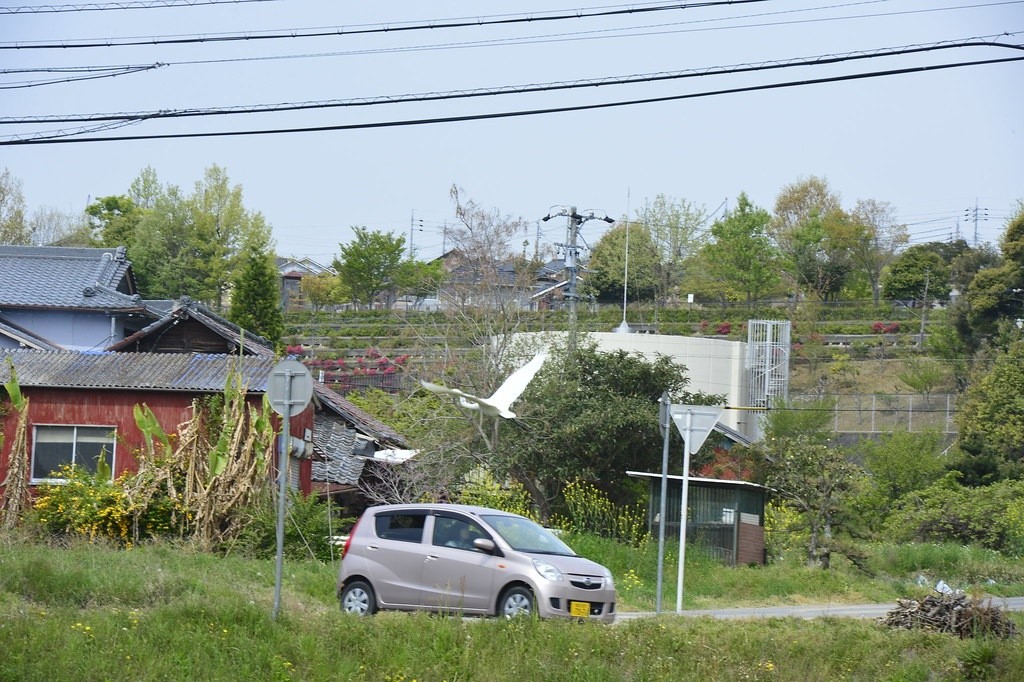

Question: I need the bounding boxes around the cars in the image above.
[335,502,617,629]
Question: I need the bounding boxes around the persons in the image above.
[445,521,474,549]
